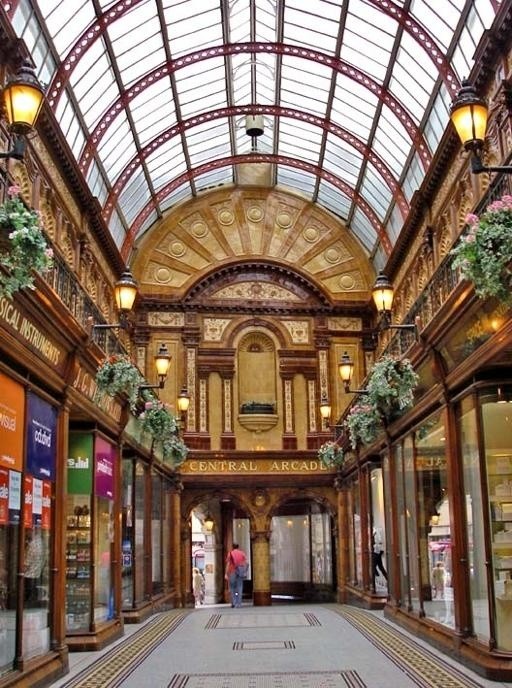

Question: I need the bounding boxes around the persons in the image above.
[224,542,248,608]
[371,526,387,595]
[432,562,447,599]
[192,567,205,606]
[25,527,46,610]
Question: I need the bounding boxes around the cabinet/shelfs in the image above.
[67,525,91,615]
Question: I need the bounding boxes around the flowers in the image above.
[139,400,189,466]
[316,395,381,468]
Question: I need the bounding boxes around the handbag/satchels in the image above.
[235,565,247,579]
